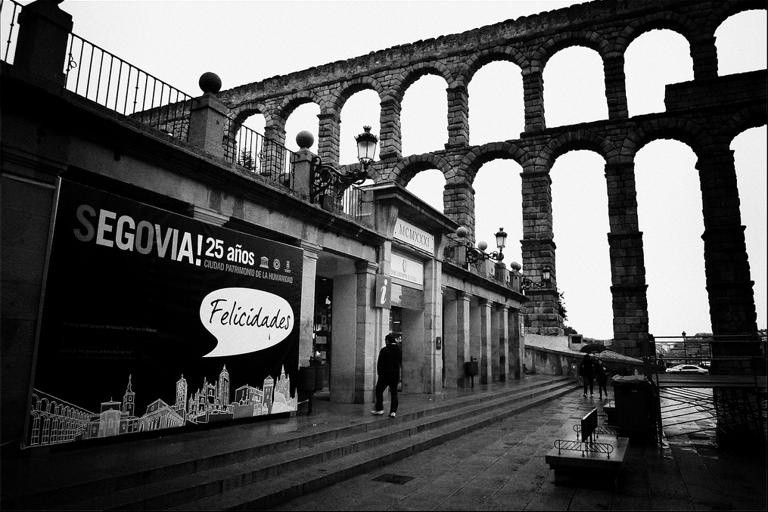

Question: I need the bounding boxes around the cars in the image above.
[666,364,708,374]
[698,360,711,369]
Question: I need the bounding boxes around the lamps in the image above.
[468,226,509,264]
[330,124,379,199]
[519,265,552,291]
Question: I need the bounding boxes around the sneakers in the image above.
[370,410,384,415]
[391,412,395,417]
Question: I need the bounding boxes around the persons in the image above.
[369,332,402,419]
[578,353,596,398]
[593,358,609,400]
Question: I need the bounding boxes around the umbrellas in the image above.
[579,342,606,354]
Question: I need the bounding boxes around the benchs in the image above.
[542,406,631,492]
